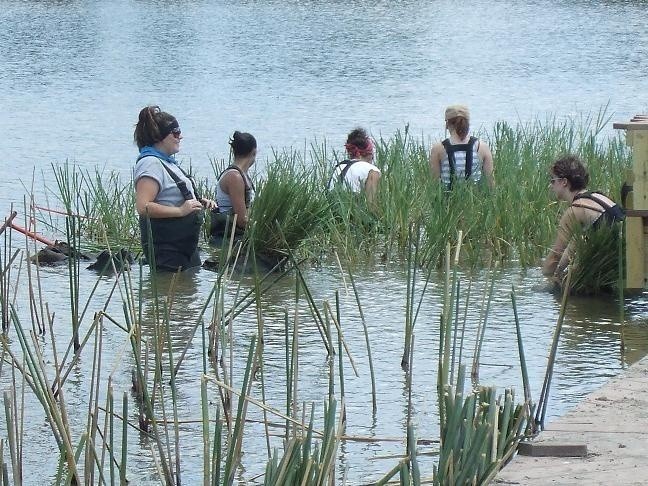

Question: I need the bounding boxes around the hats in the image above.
[443,103,472,122]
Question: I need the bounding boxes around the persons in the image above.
[325,127,382,233]
[210,130,258,239]
[541,155,624,277]
[132,105,219,274]
[430,104,497,200]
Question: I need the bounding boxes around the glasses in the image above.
[168,126,183,139]
[550,176,567,184]
